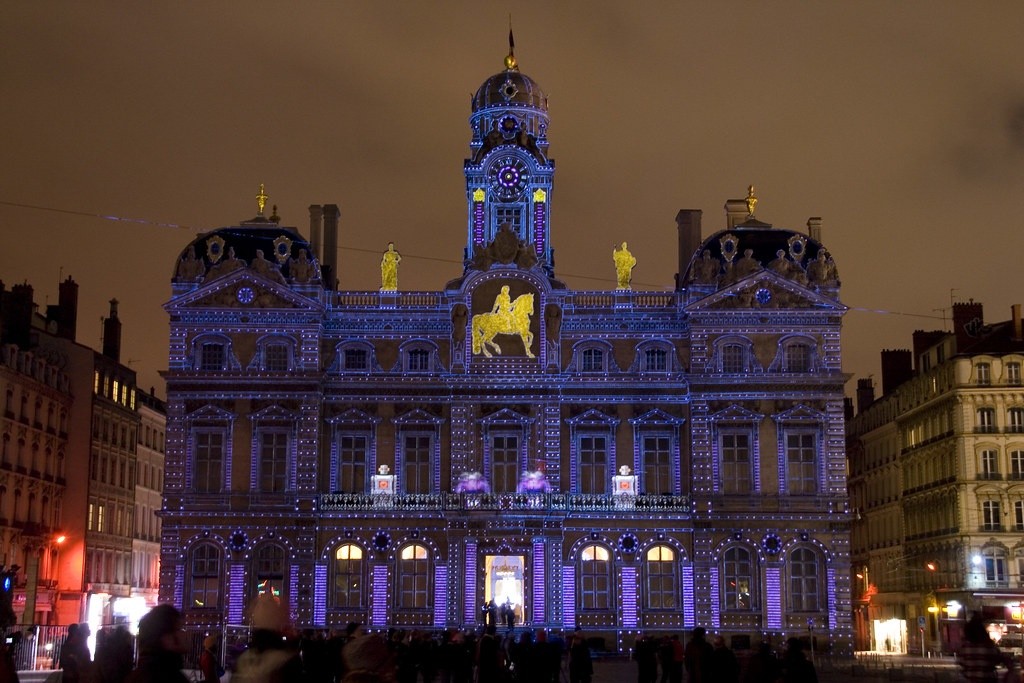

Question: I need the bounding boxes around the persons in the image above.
[955,611,1016,683]
[199,636,225,683]
[52,594,817,683]
